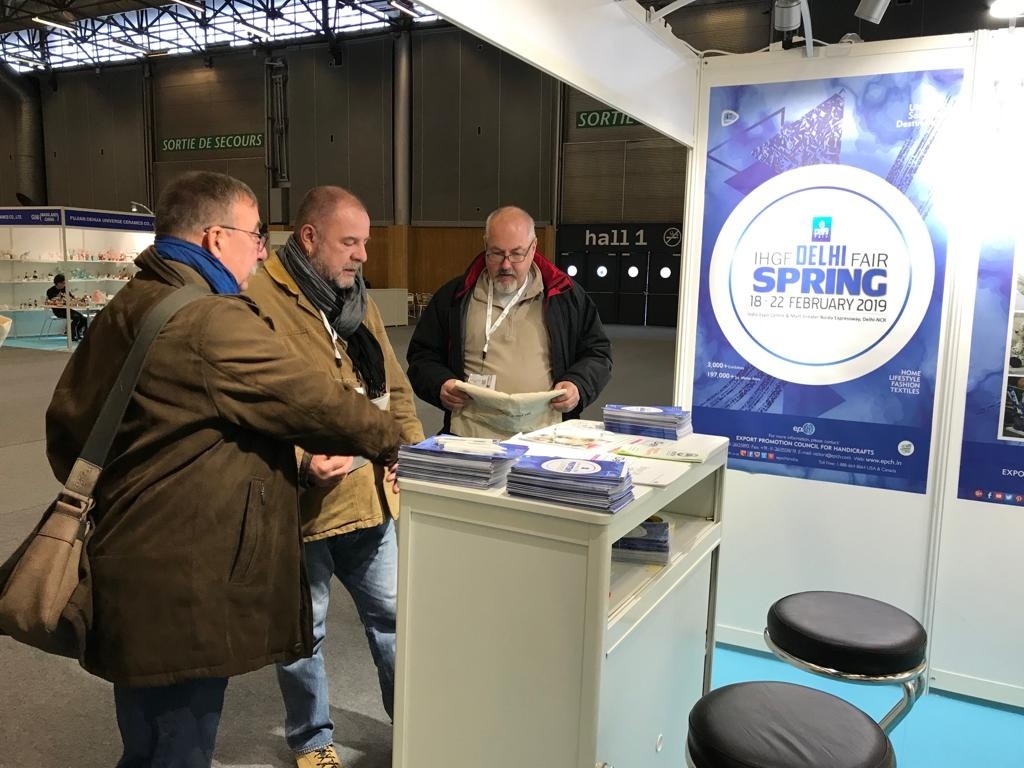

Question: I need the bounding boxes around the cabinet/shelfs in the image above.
[0,259,137,312]
[392,419,729,768]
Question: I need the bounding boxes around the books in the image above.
[601,403,693,440]
[612,521,671,565]
[396,434,635,515]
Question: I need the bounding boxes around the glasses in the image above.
[486,240,534,264]
[205,226,268,252]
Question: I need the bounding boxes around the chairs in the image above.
[38,296,80,341]
[418,293,434,317]
[407,293,416,318]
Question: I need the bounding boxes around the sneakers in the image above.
[294,744,344,768]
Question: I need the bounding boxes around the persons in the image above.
[407,207,613,442]
[46,274,87,341]
[243,185,426,768]
[46,171,407,768]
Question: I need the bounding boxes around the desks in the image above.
[41,306,101,328]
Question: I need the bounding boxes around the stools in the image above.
[764,591,925,736]
[685,681,898,768]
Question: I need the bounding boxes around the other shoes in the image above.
[72,331,77,340]
[78,329,84,339]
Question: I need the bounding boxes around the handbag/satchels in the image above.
[0,493,96,659]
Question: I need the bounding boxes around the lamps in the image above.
[171,0,206,13]
[774,0,801,31]
[31,15,78,31]
[130,201,153,215]
[237,21,270,38]
[387,0,422,17]
[111,39,147,53]
[855,0,890,24]
[10,55,46,66]
